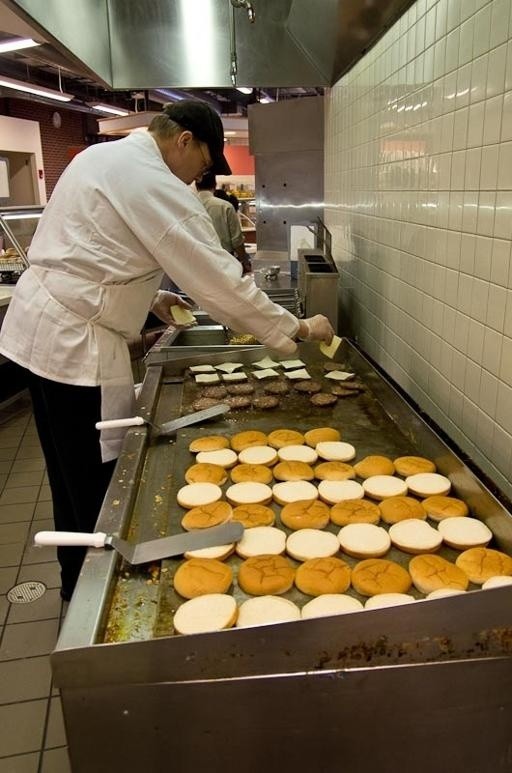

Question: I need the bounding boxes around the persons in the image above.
[190,165,255,274]
[0,97,338,600]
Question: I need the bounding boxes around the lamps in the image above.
[0,31,141,117]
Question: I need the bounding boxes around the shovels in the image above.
[95,403,231,434]
[34,521,244,566]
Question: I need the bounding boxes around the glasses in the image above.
[197,144,214,176]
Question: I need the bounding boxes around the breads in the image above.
[181,502,232,530]
[378,496,427,523]
[226,482,273,506]
[185,463,227,487]
[315,441,356,462]
[340,380,370,391]
[424,588,471,601]
[226,384,254,396]
[201,386,227,400]
[331,499,381,527]
[270,429,305,446]
[280,500,331,530]
[331,386,359,399]
[173,559,235,598]
[231,431,269,451]
[353,455,396,479]
[422,495,468,523]
[303,428,340,448]
[319,479,364,505]
[364,594,418,616]
[231,463,273,486]
[302,594,364,620]
[277,444,318,465]
[186,359,311,385]
[274,461,314,481]
[273,482,319,507]
[314,460,356,480]
[176,481,223,509]
[252,396,279,410]
[393,456,436,476]
[184,544,234,563]
[238,446,278,466]
[362,475,408,500]
[454,547,512,586]
[287,528,340,561]
[223,396,251,411]
[322,361,347,371]
[189,436,230,453]
[264,381,290,395]
[196,448,238,469]
[327,375,354,382]
[351,557,412,593]
[172,593,237,638]
[192,397,220,411]
[230,504,277,528]
[482,575,512,590]
[236,595,301,628]
[338,523,390,557]
[233,526,287,559]
[409,552,469,594]
[405,472,451,497]
[439,516,493,552]
[293,380,323,394]
[238,554,294,596]
[389,518,443,555]
[295,557,351,594]
[309,392,338,408]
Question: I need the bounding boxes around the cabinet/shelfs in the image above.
[0,303,36,405]
[0,203,48,286]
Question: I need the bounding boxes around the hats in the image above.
[162,99,232,176]
